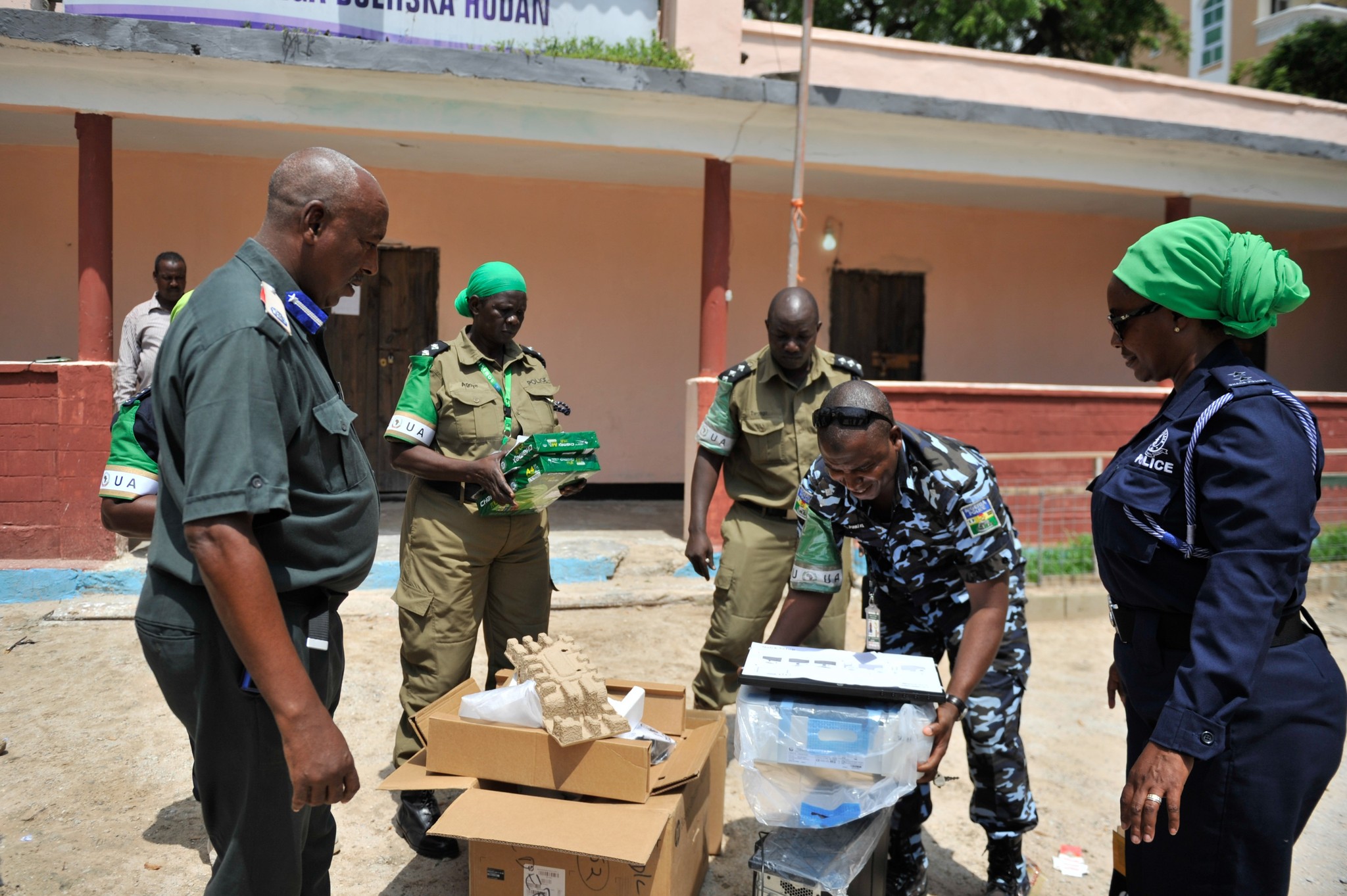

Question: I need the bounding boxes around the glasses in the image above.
[1107,302,1163,341]
[812,406,893,429]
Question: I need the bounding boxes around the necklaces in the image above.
[493,346,505,360]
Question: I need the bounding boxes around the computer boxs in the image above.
[746,780,896,896]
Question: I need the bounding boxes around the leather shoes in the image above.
[395,790,460,860]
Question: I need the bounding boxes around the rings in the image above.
[1145,794,1161,804]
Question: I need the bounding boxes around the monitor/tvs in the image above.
[738,642,946,704]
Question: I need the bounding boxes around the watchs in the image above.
[944,693,968,722]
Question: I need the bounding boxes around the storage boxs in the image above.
[374,669,730,896]
[469,431,601,518]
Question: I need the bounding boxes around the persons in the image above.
[736,379,1042,896]
[135,144,388,895]
[112,252,186,413]
[1084,215,1347,896]
[99,289,195,540]
[383,261,586,861]
[684,286,856,712]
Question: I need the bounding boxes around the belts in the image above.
[738,499,798,520]
[422,477,481,503]
[277,583,348,613]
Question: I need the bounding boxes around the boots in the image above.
[884,826,929,896]
[982,832,1031,896]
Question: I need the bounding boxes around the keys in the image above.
[934,774,959,787]
